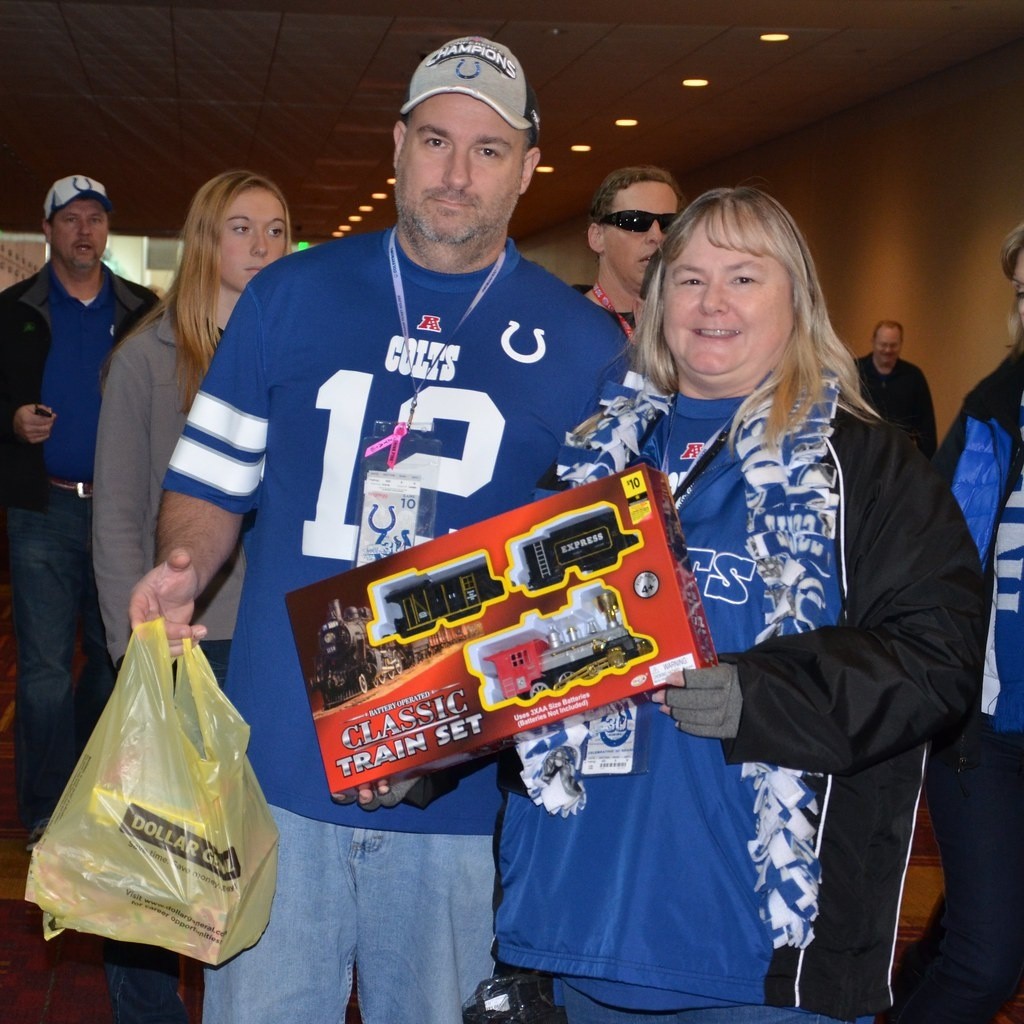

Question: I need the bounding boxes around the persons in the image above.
[130,37,632,1023]
[0,175,162,852]
[333,189,983,1024]
[587,165,679,339]
[853,320,938,458]
[886,222,1024,1024]
[91,171,293,1024]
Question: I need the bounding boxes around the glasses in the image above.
[597,210,678,234]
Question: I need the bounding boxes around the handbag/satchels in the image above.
[25,617,280,966]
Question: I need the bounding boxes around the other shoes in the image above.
[26,820,49,850]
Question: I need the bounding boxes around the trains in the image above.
[312,597,408,709]
[480,588,646,702]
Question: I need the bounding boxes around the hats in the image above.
[399,34,539,149]
[43,175,114,221]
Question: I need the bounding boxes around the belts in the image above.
[44,476,91,499]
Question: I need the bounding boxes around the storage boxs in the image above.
[87,779,225,848]
[24,828,280,967]
[282,462,720,794]
